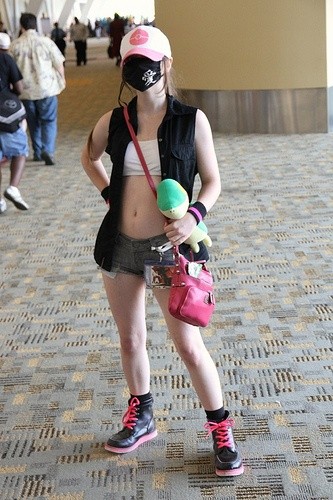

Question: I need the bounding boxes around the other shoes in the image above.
[84,59,86,64]
[41,150,55,165]
[34,157,41,161]
[77,63,81,65]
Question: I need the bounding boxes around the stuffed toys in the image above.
[157,178,212,253]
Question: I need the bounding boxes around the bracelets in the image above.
[101,186,109,204]
[188,202,207,226]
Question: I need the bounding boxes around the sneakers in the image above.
[0,199,7,213]
[4,186,30,210]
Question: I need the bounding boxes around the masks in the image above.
[123,58,165,92]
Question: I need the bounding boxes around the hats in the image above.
[120,26,171,68]
[0,32,11,50]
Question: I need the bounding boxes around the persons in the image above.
[108,13,125,66]
[0,32,29,212]
[80,25,243,476]
[8,14,66,165]
[50,23,72,54]
[70,18,88,65]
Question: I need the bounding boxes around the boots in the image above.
[203,410,244,476]
[105,398,158,454]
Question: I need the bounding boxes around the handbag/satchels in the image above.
[0,86,28,133]
[108,42,115,58]
[169,253,215,327]
